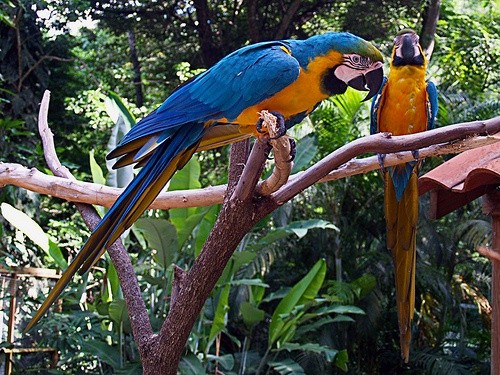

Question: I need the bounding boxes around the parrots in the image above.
[20,32,383,334]
[371,27,439,367]
